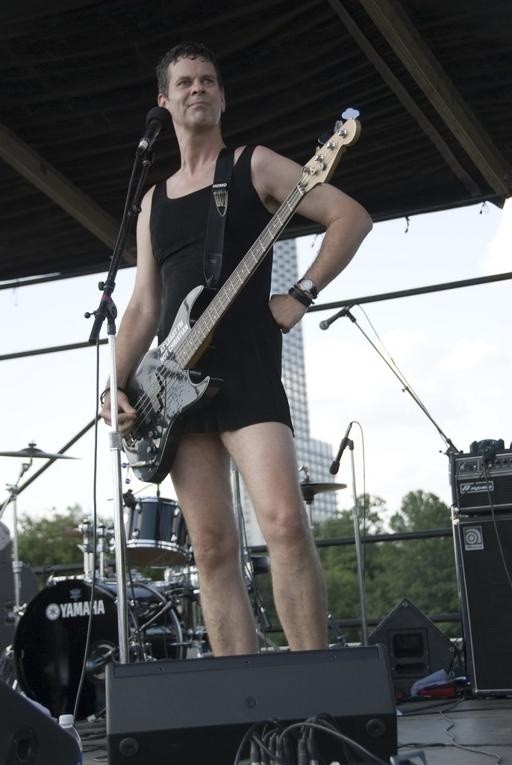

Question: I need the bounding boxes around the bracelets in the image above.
[288,287,313,309]
[293,282,316,304]
[100,387,126,405]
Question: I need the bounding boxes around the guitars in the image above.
[121,108,361,484]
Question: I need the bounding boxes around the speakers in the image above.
[103,642,399,765]
[451,511,512,698]
[355,598,459,702]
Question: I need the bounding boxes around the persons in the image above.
[100,42,374,656]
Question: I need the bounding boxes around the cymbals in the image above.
[0,440,79,459]
[300,479,347,499]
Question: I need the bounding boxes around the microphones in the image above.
[328,423,352,476]
[318,304,354,329]
[135,106,171,156]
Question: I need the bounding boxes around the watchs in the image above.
[298,276,319,298]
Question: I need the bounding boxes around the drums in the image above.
[5,574,195,726]
[121,496,194,567]
